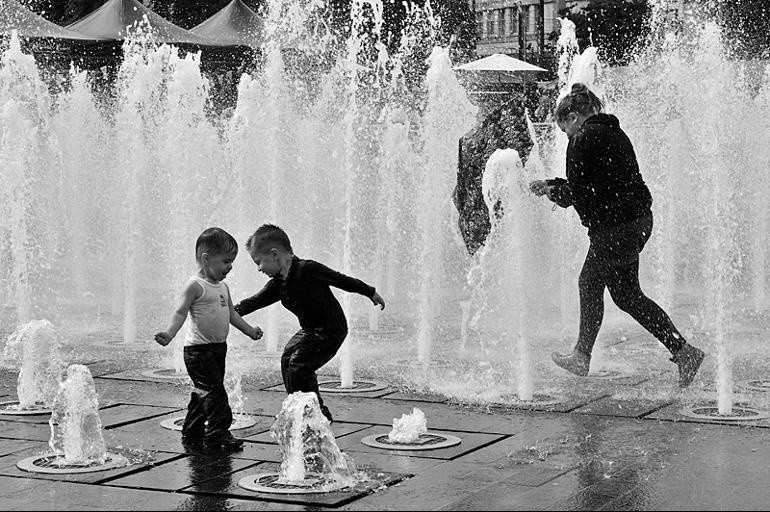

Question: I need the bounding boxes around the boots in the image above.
[668,343,705,388]
[550,346,593,379]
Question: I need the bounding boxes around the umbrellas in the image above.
[447,51,553,84]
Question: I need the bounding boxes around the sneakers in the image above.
[201,436,245,450]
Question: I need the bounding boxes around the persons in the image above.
[524,81,709,390]
[232,221,389,427]
[148,225,264,452]
